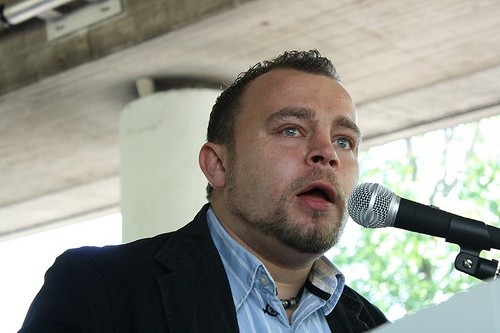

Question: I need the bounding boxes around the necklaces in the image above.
[279,296,299,308]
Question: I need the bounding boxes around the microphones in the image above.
[347,182,500,251]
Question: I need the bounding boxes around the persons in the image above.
[16,50,390,333]
